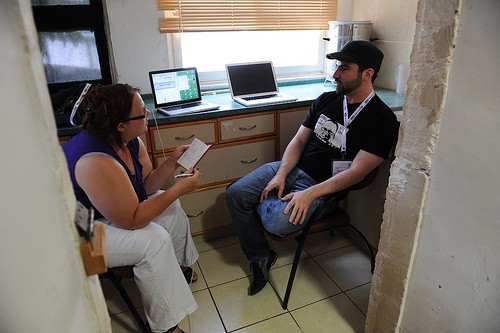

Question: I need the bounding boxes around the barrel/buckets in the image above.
[324,21,379,86]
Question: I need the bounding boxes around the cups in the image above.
[396,64,409,94]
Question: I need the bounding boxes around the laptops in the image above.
[148,67,220,116]
[225,61,298,107]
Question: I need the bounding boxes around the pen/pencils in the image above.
[175,173,202,178]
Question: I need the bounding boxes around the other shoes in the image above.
[181,266,198,284]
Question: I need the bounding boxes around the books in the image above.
[176,137,212,170]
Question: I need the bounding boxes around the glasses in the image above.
[125,107,147,122]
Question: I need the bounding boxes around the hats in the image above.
[326,41,385,72]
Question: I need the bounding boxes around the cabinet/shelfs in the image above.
[145,81,407,237]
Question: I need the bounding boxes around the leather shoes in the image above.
[248,250,278,296]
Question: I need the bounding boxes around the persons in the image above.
[226,40,399,297]
[56,82,200,333]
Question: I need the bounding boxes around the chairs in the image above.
[77,223,152,333]
[252,119,400,311]
